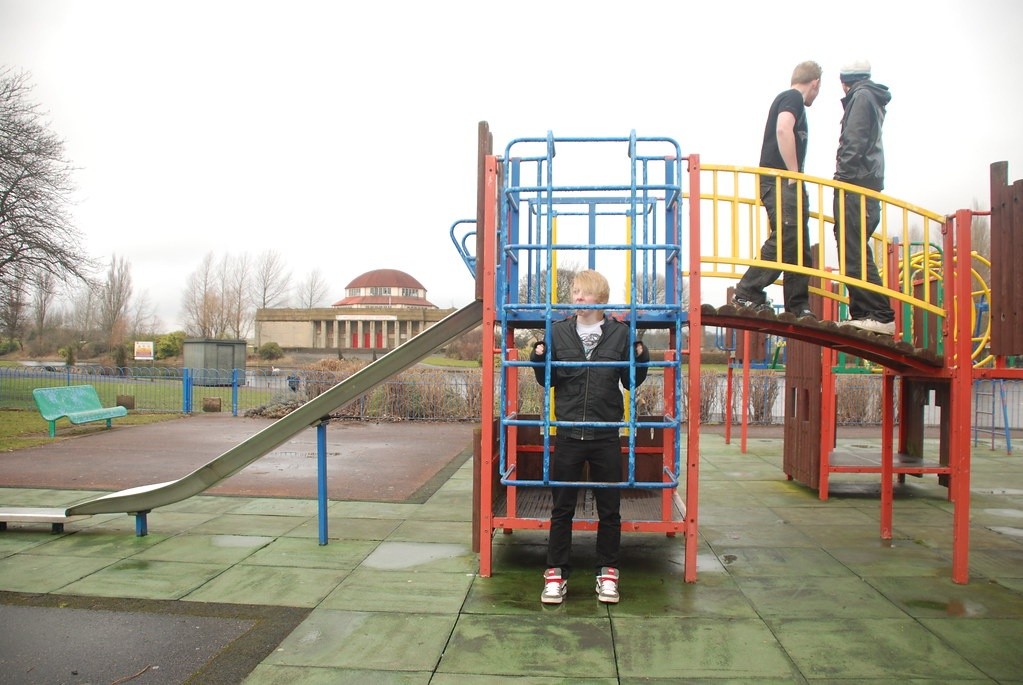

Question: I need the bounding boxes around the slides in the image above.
[64,294,483,523]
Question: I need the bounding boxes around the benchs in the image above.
[32,384,129,437]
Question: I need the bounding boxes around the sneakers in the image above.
[796,309,815,319]
[832,319,860,329]
[538,567,568,603]
[736,297,773,314]
[845,318,896,336]
[593,567,621,603]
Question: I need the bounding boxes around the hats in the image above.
[840,52,871,83]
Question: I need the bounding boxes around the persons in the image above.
[529,268,650,602]
[732,60,823,318]
[831,60,896,335]
